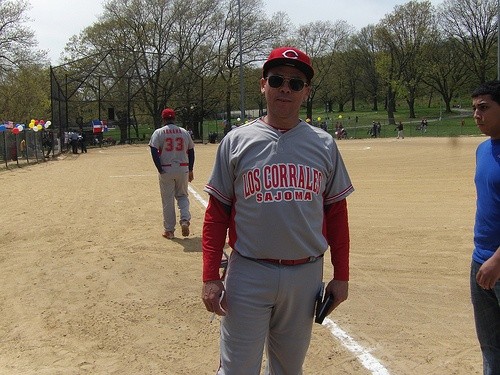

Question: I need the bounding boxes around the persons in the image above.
[394,120,404,139]
[370,120,382,138]
[420,119,428,133]
[470,80,500,375]
[185,119,195,140]
[148,108,196,240]
[0,128,116,161]
[208,120,250,144]
[202,48,355,375]
[313,118,348,140]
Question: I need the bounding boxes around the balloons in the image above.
[224,119,228,125]
[338,115,342,118]
[0,119,52,134]
[237,117,241,122]
[318,117,322,122]
[306,118,311,123]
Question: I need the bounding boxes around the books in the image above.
[315,282,334,324]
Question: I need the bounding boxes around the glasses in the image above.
[265,75,307,91]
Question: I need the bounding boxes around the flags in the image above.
[93,119,108,134]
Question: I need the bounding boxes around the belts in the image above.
[257,253,324,265]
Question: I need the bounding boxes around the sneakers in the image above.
[162,231,174,239]
[181,224,190,237]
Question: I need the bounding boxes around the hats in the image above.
[263,48,314,80]
[160,108,175,122]
